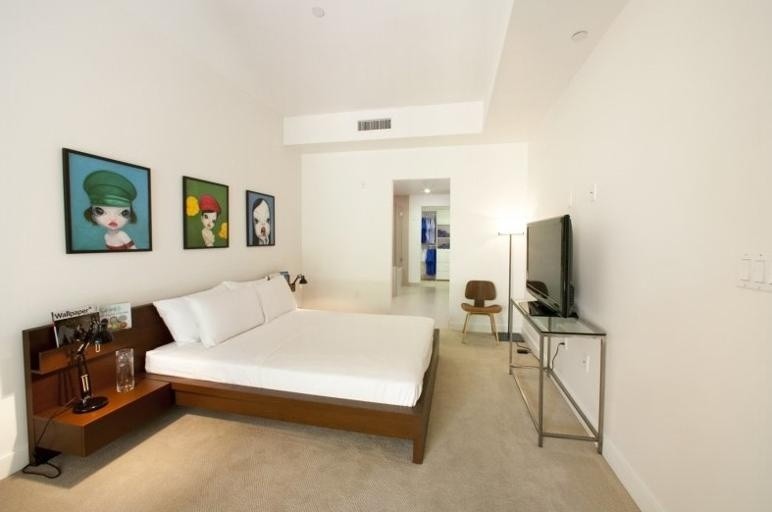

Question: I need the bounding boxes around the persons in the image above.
[82,169,139,251]
[198,193,222,247]
[252,197,272,246]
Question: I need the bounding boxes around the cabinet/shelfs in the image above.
[436,210,449,281]
[31,337,171,456]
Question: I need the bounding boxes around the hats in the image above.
[83,170,137,207]
[198,194,221,214]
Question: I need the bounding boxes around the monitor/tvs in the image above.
[518,214,579,318]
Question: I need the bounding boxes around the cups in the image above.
[115,348,135,393]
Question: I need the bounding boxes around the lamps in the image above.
[72,320,115,414]
[289,274,308,291]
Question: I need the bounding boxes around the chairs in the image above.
[461,280,502,345]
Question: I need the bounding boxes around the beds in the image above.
[143,271,441,465]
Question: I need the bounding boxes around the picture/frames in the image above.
[182,175,229,250]
[61,147,153,254]
[245,189,279,247]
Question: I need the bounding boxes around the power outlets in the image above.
[558,337,567,351]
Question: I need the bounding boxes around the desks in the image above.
[509,298,608,455]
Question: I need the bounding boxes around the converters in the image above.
[517,349,528,353]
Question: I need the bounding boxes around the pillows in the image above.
[154,273,297,349]
